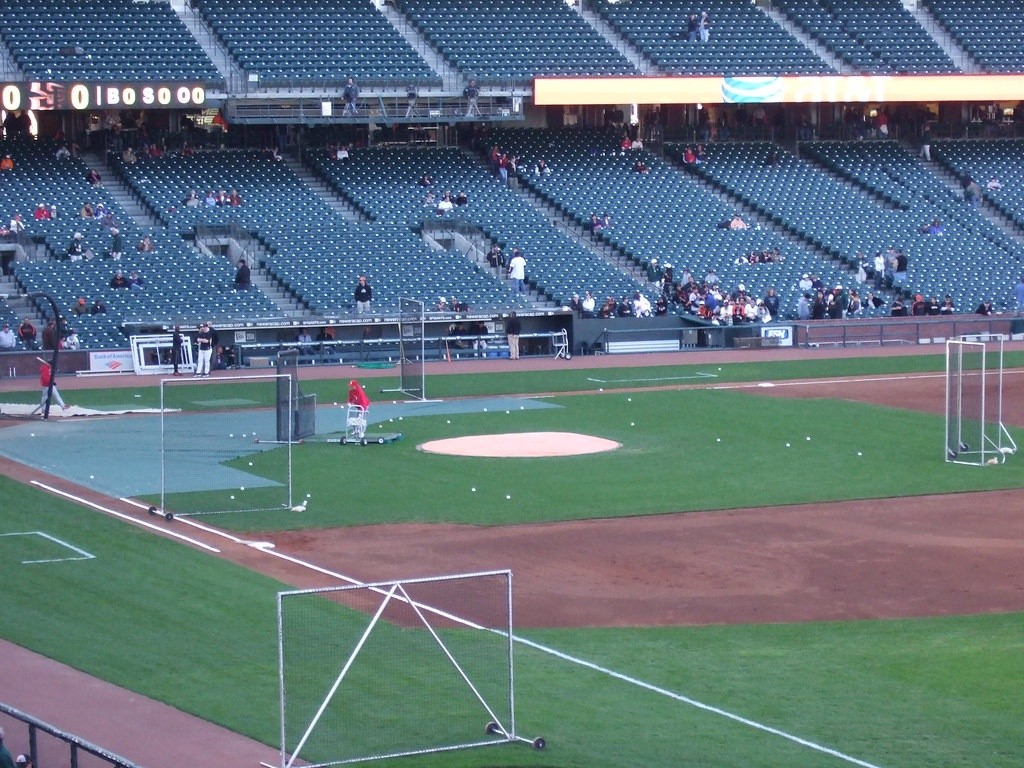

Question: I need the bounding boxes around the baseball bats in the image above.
[36,356,59,371]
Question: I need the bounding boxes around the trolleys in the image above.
[552,328,572,361]
[339,404,369,446]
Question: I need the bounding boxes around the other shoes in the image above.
[351,431,358,435]
[359,433,365,438]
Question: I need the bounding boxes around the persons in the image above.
[962,171,1001,208]
[486,243,526,295]
[298,326,338,364]
[1014,279,1024,310]
[39,359,69,416]
[435,296,521,359]
[172,323,243,377]
[340,77,358,108]
[570,212,993,323]
[354,276,372,313]
[688,11,711,42]
[0,109,406,260]
[347,380,370,439]
[236,259,251,290]
[110,268,144,288]
[418,123,549,217]
[0,315,80,351]
[463,79,480,100]
[73,298,106,314]
[604,103,1024,173]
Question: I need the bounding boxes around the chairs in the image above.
[588,0,841,77]
[661,141,1024,315]
[384,0,648,86]
[0,0,228,90]
[189,0,445,89]
[770,0,964,74]
[921,0,1024,75]
[0,137,688,366]
[475,124,914,322]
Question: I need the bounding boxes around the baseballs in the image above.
[446,420,451,424]
[716,438,721,442]
[472,488,477,492]
[718,368,721,370]
[362,385,366,388]
[482,406,525,415]
[31,433,35,437]
[858,452,862,456]
[784,437,811,448]
[89,474,95,480]
[332,400,404,428]
[506,494,511,500]
[227,430,257,502]
[598,387,635,428]
[302,494,311,506]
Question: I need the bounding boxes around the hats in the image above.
[916,294,922,301]
[441,297,446,303]
[349,380,358,387]
[200,323,209,328]
[360,277,366,281]
[79,298,85,304]
